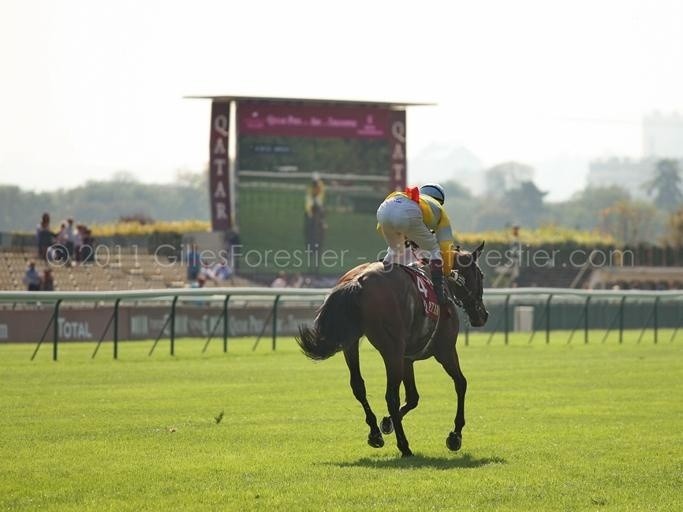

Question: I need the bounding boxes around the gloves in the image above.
[446,267,467,288]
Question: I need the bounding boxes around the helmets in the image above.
[420,184,445,206]
[309,169,321,181]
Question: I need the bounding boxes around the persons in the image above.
[197,262,214,285]
[375,181,459,304]
[303,180,328,248]
[268,270,289,288]
[42,265,54,291]
[290,270,305,288]
[35,211,96,263]
[183,244,201,287]
[208,260,232,282]
[20,262,42,291]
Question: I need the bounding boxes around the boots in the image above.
[429,267,450,306]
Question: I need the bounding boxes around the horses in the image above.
[295,238,490,456]
